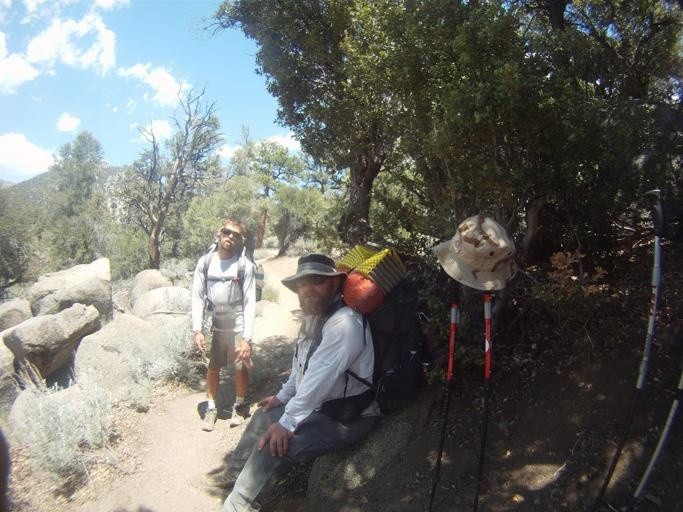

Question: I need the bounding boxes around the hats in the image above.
[281,253,348,293]
[433,215,518,291]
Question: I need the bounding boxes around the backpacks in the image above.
[204,239,262,303]
[314,280,422,412]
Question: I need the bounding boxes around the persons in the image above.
[191,221,258,431]
[205,252,382,512]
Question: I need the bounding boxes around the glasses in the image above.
[295,274,337,288]
[222,228,243,241]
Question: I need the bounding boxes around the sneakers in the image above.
[230,402,248,426]
[206,469,242,487]
[202,407,218,431]
